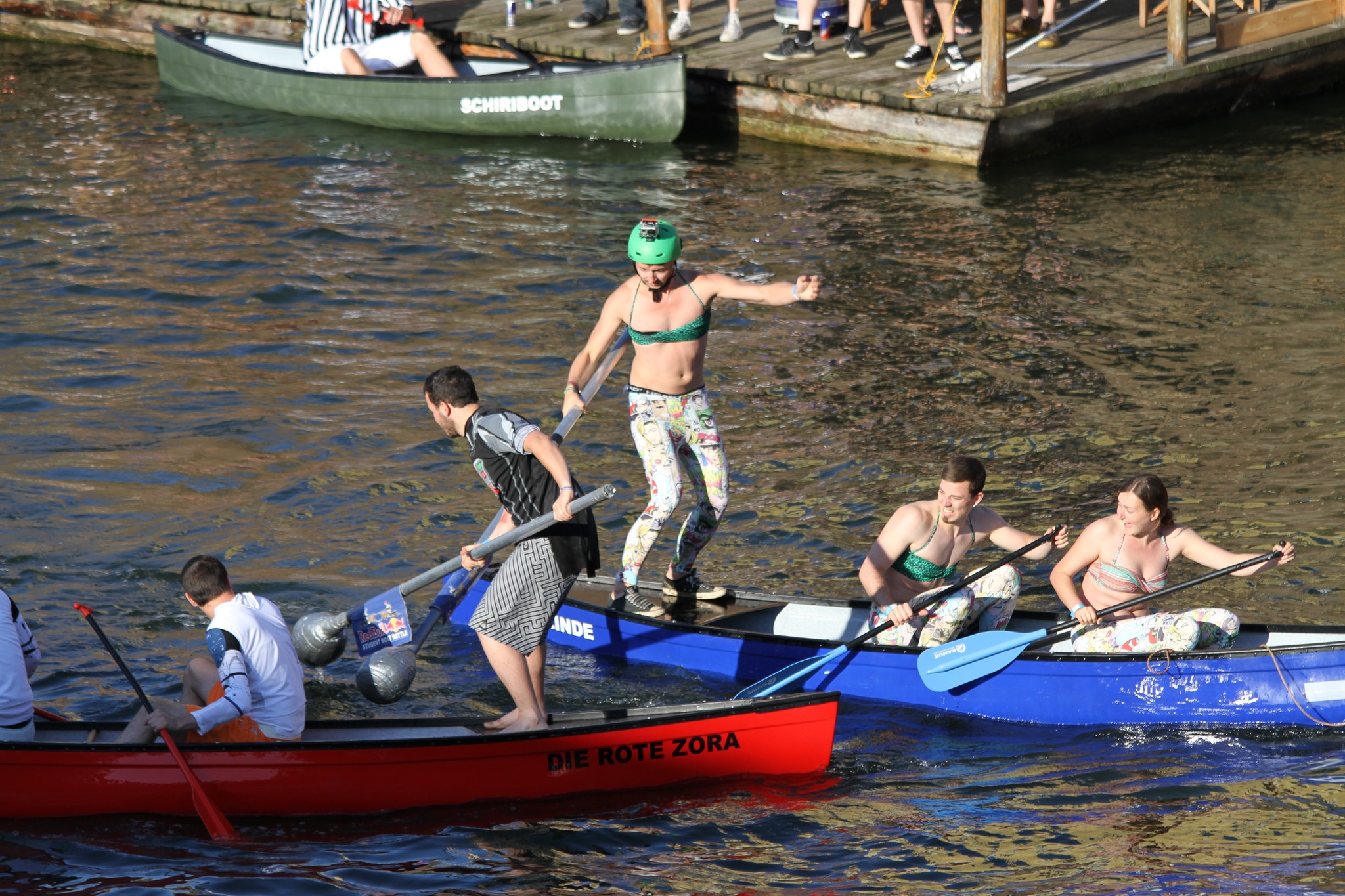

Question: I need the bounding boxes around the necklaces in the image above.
[650,287,675,301]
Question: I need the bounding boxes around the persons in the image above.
[0,587,40,744]
[423,365,601,735]
[566,0,1060,71]
[859,456,1069,647]
[298,0,460,78]
[112,553,308,749]
[1050,473,1295,653]
[561,218,822,618]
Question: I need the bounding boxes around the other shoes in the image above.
[1005,14,1042,40]
[1037,22,1060,49]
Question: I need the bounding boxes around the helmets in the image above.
[627,219,681,264]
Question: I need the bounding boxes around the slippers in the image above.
[910,16,933,38]
[954,17,974,37]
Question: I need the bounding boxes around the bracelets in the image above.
[883,603,898,616]
[1070,603,1085,618]
[482,539,491,545]
[559,486,578,496]
[563,381,581,396]
[792,285,802,301]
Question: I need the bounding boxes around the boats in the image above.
[431,548,1345,737]
[0,690,852,826]
[150,19,688,149]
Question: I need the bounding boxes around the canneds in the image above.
[819,11,831,40]
[506,0,516,27]
[525,0,533,10]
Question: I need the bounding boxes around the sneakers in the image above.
[763,37,817,60]
[944,43,970,71]
[567,11,606,28]
[842,33,870,59]
[895,43,934,69]
[616,18,644,35]
[667,8,692,41]
[661,568,727,600]
[719,9,744,43]
[606,585,666,618]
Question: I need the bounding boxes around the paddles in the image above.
[74,603,239,842]
[734,522,1063,700]
[34,705,68,721]
[916,540,1286,693]
